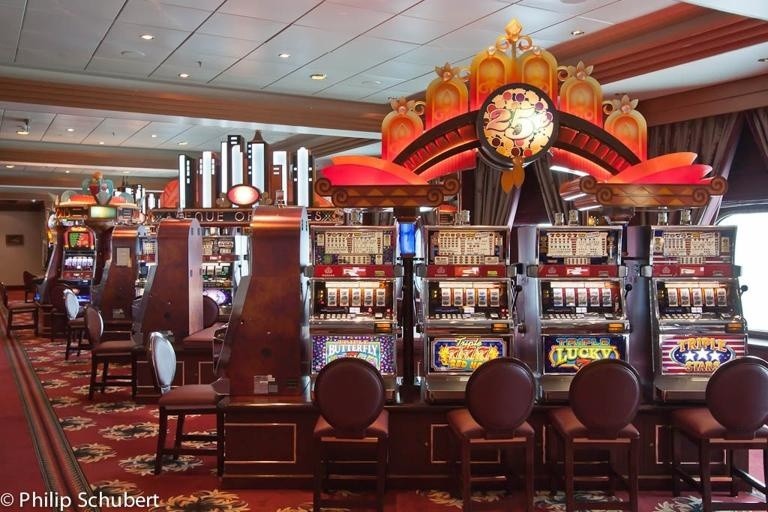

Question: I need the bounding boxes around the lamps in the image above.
[15,125,31,135]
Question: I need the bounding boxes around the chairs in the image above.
[145,328,226,478]
[448,354,644,512]
[671,355,768,508]
[0,269,41,343]
[50,281,135,401]
[304,354,394,512]
